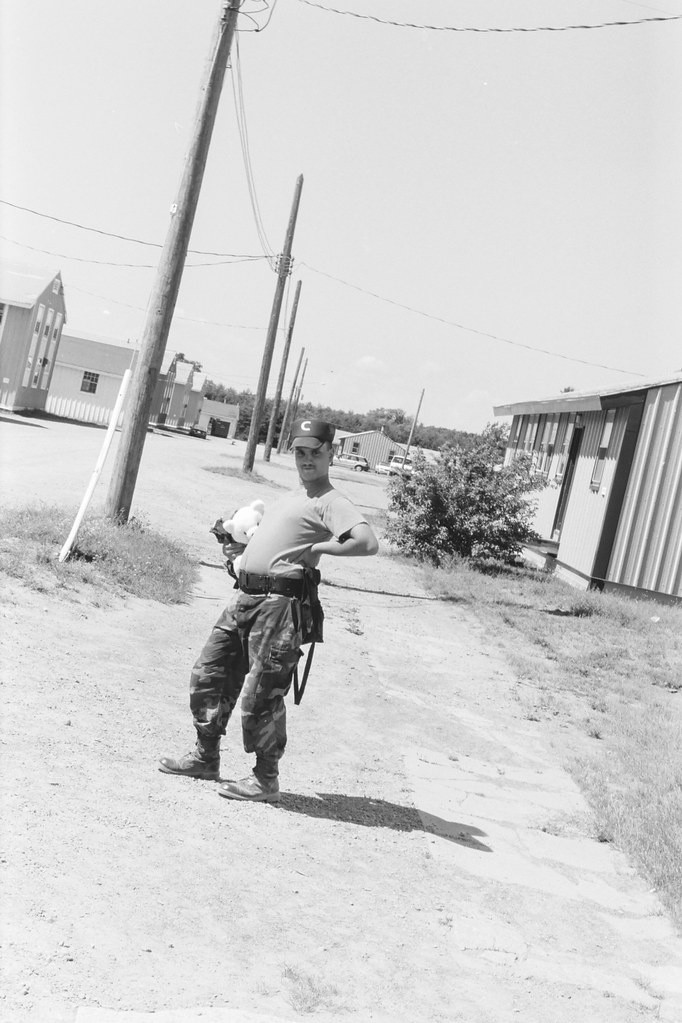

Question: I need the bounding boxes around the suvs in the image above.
[329,453,370,472]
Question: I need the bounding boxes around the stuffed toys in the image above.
[222,499,266,579]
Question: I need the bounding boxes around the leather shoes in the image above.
[158,750,220,780]
[218,773,280,803]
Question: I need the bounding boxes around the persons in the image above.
[158,419,379,803]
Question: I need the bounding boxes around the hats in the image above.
[290,420,335,449]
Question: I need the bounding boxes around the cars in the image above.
[374,454,412,478]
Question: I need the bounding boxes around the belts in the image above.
[239,572,302,594]
